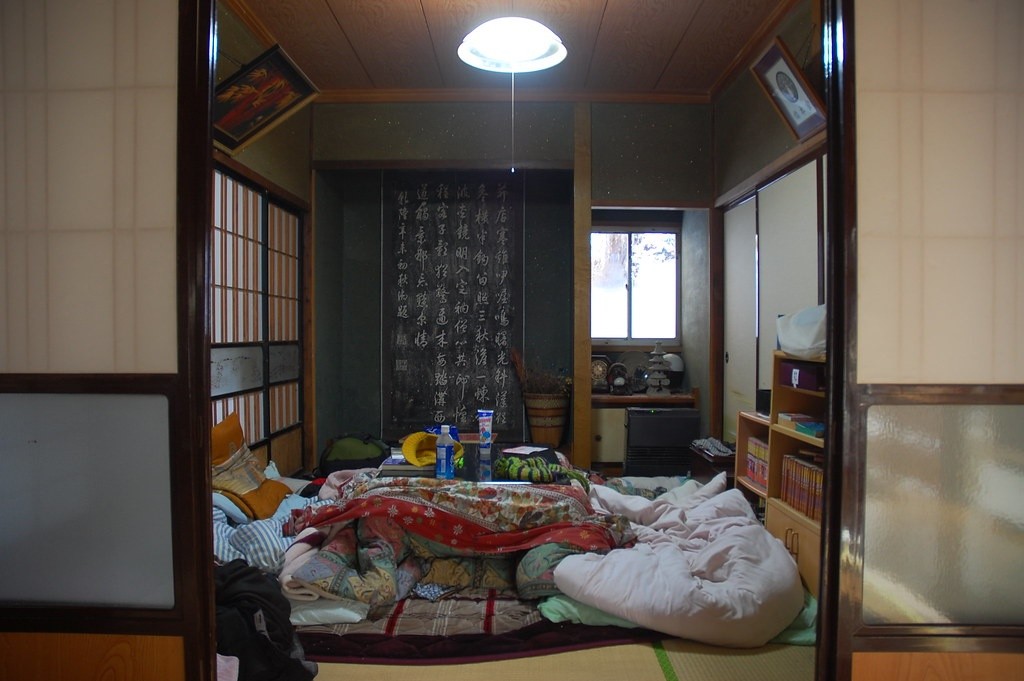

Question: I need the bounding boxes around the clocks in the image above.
[591,355,612,392]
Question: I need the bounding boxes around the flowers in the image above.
[511,347,573,398]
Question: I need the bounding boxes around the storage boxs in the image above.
[780,362,819,391]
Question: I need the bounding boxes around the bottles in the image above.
[450,427,460,442]
[435,425,455,480]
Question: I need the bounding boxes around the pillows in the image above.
[210,412,293,519]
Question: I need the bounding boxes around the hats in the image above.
[403,432,465,467]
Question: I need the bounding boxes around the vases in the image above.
[523,392,568,448]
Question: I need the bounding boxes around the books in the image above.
[382,447,436,471]
[780,448,823,523]
[746,437,769,490]
[777,412,825,438]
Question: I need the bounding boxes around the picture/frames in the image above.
[213,43,320,157]
[747,35,827,145]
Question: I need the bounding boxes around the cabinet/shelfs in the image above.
[591,386,700,466]
[734,349,825,601]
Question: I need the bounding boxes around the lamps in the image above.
[456,17,568,173]
